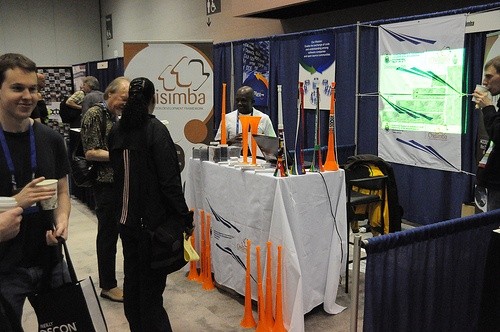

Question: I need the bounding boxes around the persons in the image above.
[0,53,79,332]
[80,78,131,303]
[65,88,89,199]
[82,74,105,117]
[35,73,49,127]
[471,55,500,213]
[213,86,279,158]
[107,77,194,332]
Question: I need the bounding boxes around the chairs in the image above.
[339,155,389,292]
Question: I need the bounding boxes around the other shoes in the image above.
[100,287,123,302]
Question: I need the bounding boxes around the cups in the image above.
[36,179,58,210]
[471,84,490,102]
[0,196,17,215]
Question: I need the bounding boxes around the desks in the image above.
[182,153,347,320]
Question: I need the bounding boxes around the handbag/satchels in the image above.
[60,95,82,123]
[71,153,97,194]
[32,236,109,332]
